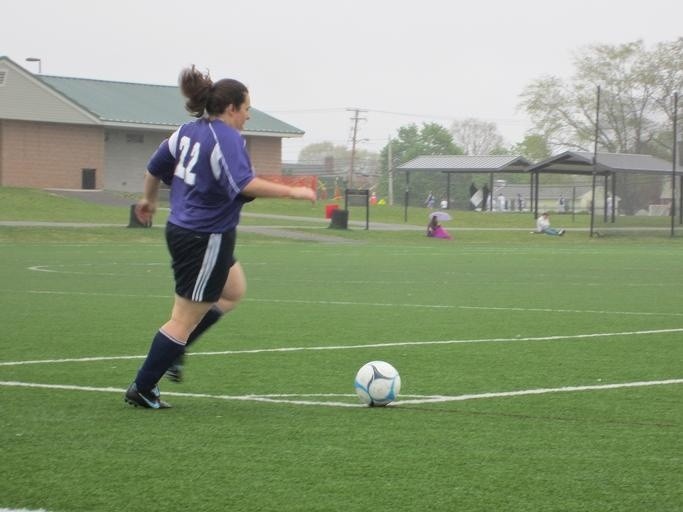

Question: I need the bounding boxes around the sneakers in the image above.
[167,353,183,382]
[124,382,172,408]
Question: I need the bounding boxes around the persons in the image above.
[124,66,318,410]
[536,212,565,236]
[559,194,566,212]
[498,192,525,212]
[423,190,435,209]
[607,194,612,215]
[482,183,491,210]
[469,182,478,209]
[439,198,448,209]
[426,215,451,240]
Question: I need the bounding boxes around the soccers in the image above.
[356,361,401,405]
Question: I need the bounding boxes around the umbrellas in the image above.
[428,212,453,222]
